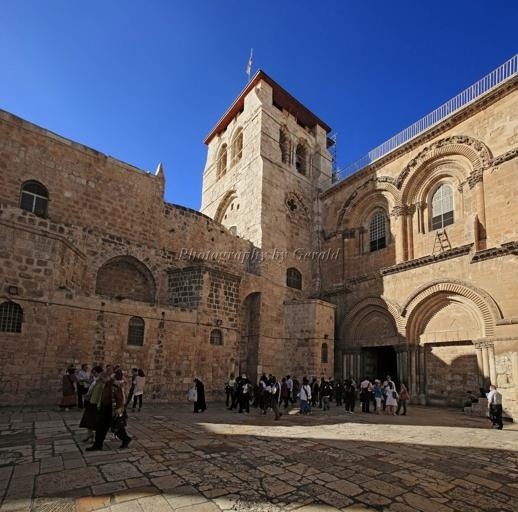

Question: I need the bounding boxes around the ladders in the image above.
[432,229,445,255]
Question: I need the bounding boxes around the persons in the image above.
[222,371,411,422]
[56,360,148,453]
[461,390,477,412]
[186,378,207,414]
[478,387,488,398]
[485,384,504,432]
